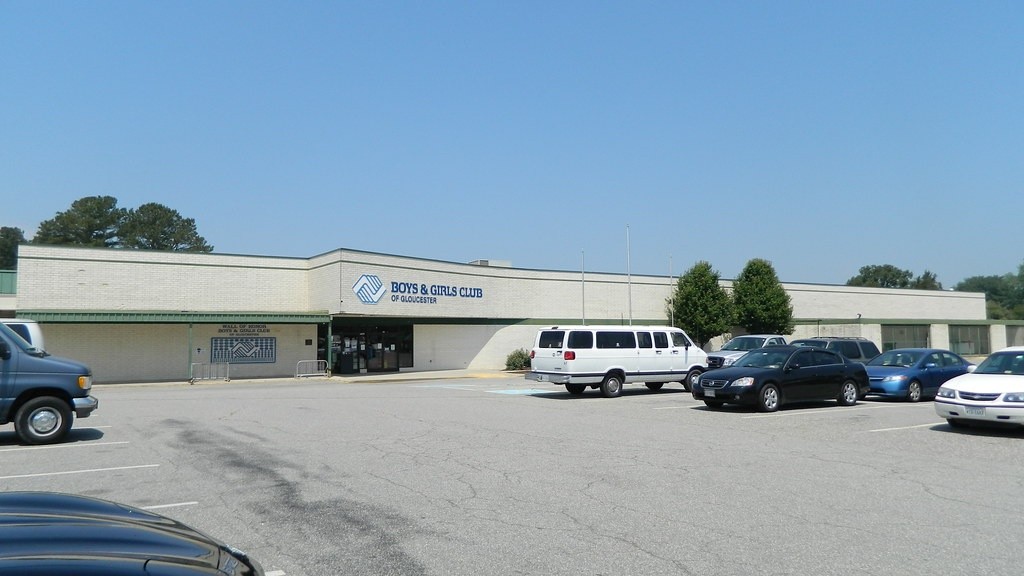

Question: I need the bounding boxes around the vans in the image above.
[524,324,709,397]
[0,318,44,351]
[0,322,99,444]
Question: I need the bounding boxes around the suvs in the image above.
[705,334,787,371]
[788,336,881,367]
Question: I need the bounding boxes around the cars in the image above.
[691,345,871,413]
[934,345,1024,429]
[0,492,265,576]
[858,347,977,402]
